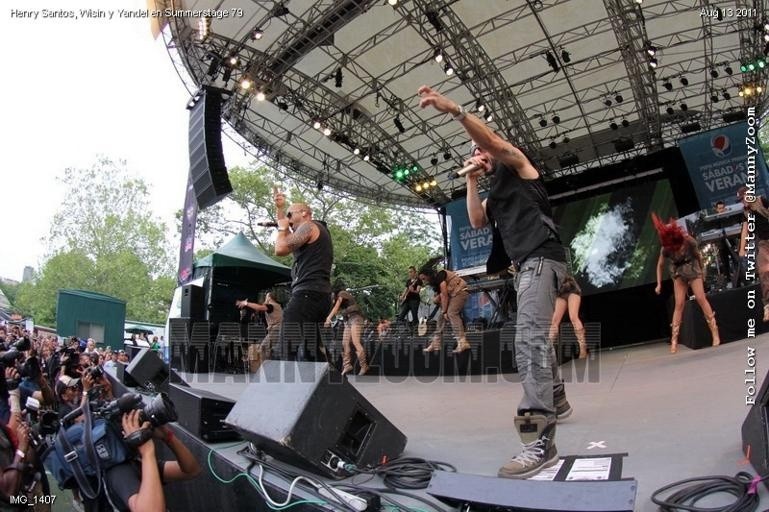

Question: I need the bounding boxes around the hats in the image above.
[55,376,81,396]
[735,187,753,203]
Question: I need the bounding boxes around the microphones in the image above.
[257,221,278,227]
[452,162,483,177]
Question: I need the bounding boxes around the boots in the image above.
[422,336,441,353]
[341,352,353,375]
[357,350,368,376]
[707,318,720,346]
[453,336,471,353]
[548,332,557,343]
[670,320,680,352]
[498,410,558,479]
[574,328,587,360]
[551,380,573,420]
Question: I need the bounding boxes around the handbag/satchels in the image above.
[42,418,127,490]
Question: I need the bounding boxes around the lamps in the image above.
[188,7,769,192]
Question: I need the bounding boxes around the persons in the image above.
[540,275,588,360]
[418,86,573,481]
[711,201,738,288]
[358,317,394,350]
[395,266,421,323]
[735,188,769,323]
[419,256,472,353]
[0,311,195,512]
[324,282,370,377]
[235,292,284,350]
[650,212,721,355]
[271,185,334,362]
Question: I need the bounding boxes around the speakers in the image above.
[168,317,209,373]
[741,370,769,492]
[181,284,205,319]
[115,344,191,393]
[167,382,243,443]
[223,358,407,480]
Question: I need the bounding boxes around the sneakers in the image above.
[762,305,769,322]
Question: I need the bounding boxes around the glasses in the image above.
[470,145,487,157]
[66,385,78,391]
[287,212,301,218]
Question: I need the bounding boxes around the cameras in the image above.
[14,356,39,378]
[8,334,31,351]
[86,364,104,381]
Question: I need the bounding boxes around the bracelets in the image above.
[277,226,287,232]
[452,105,467,122]
[244,300,248,306]
[442,311,447,315]
